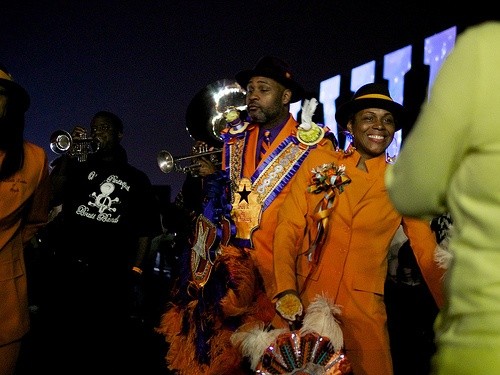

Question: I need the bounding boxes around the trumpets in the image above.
[49,128,105,162]
[158,149,222,174]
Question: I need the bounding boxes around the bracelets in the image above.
[130,261,146,276]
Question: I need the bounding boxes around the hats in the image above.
[334,83,411,132]
[234,55,307,103]
[0,69,31,114]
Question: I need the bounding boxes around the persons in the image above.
[0,68,50,375]
[48,110,155,286]
[273,82,445,375]
[191,140,231,229]
[221,54,339,328]
[386,22,500,375]
